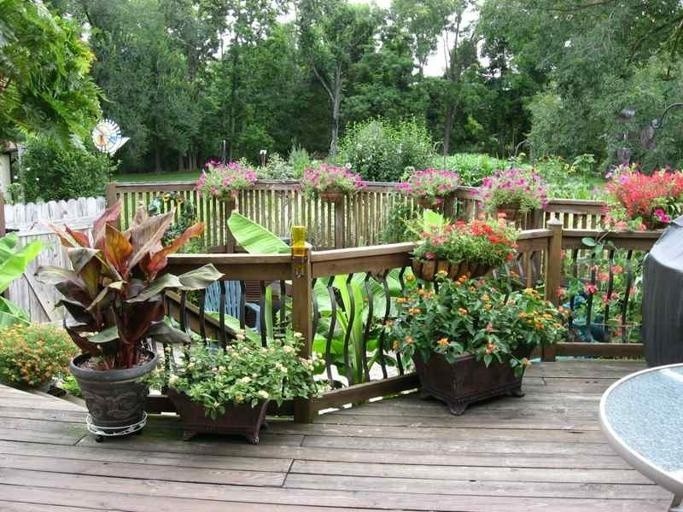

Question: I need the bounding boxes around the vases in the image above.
[160,385,271,443]
[66,350,156,435]
